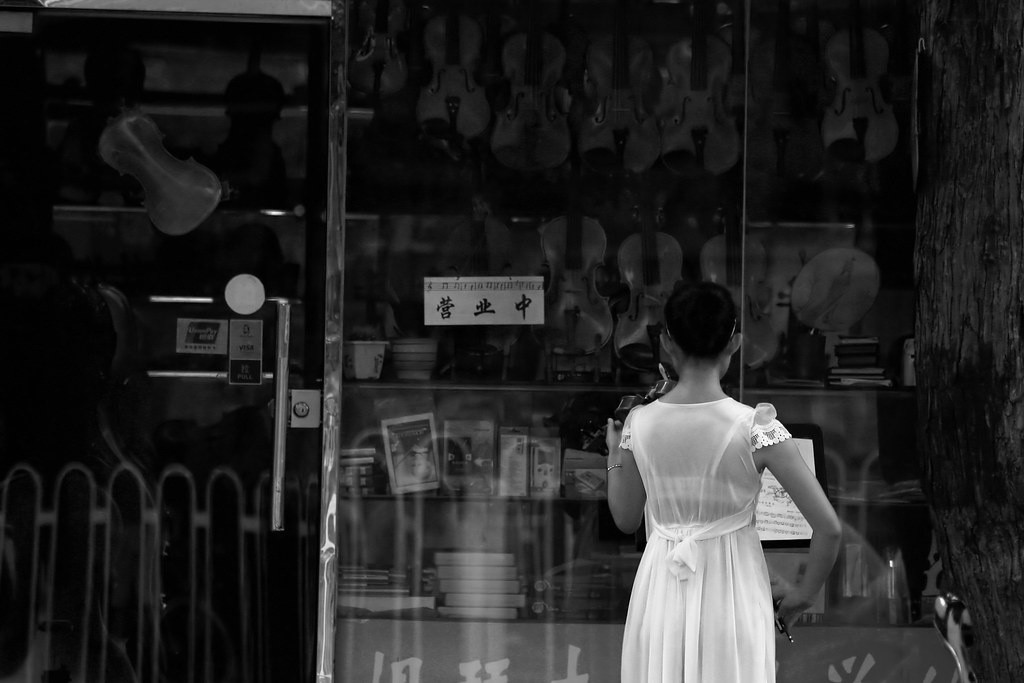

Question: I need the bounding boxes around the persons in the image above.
[602,281,845,683]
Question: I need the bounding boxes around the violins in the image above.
[85,47,223,237]
[255,2,905,377]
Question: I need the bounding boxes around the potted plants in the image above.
[343,331,390,380]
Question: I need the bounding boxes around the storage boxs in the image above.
[434,546,531,623]
[442,412,608,500]
[392,335,441,380]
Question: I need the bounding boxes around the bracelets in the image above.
[607,463,622,472]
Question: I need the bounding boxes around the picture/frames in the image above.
[380,412,442,495]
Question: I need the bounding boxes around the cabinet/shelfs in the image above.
[338,384,932,633]
